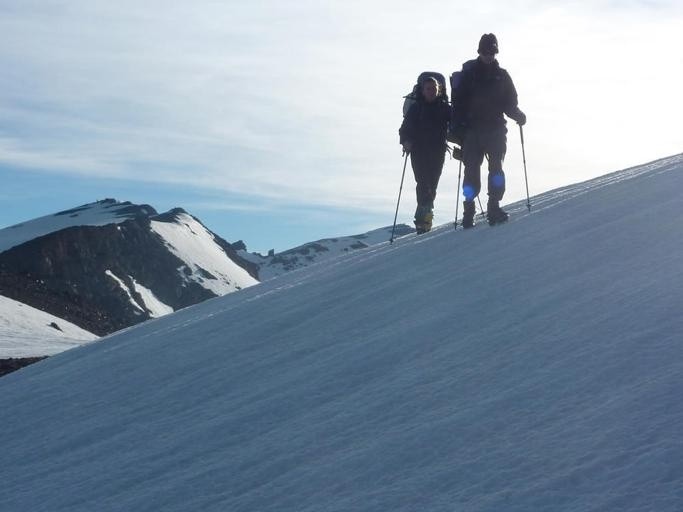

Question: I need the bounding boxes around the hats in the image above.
[478,33,499,54]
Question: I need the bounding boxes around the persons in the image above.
[398,76,451,234]
[461,34,526,227]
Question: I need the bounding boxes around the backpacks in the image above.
[446,76,463,147]
[402,71,449,118]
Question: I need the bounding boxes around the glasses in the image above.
[482,52,494,56]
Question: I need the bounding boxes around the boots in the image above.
[414,211,434,234]
[463,201,476,228]
[487,202,508,226]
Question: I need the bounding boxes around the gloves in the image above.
[403,141,412,152]
[516,113,526,126]
